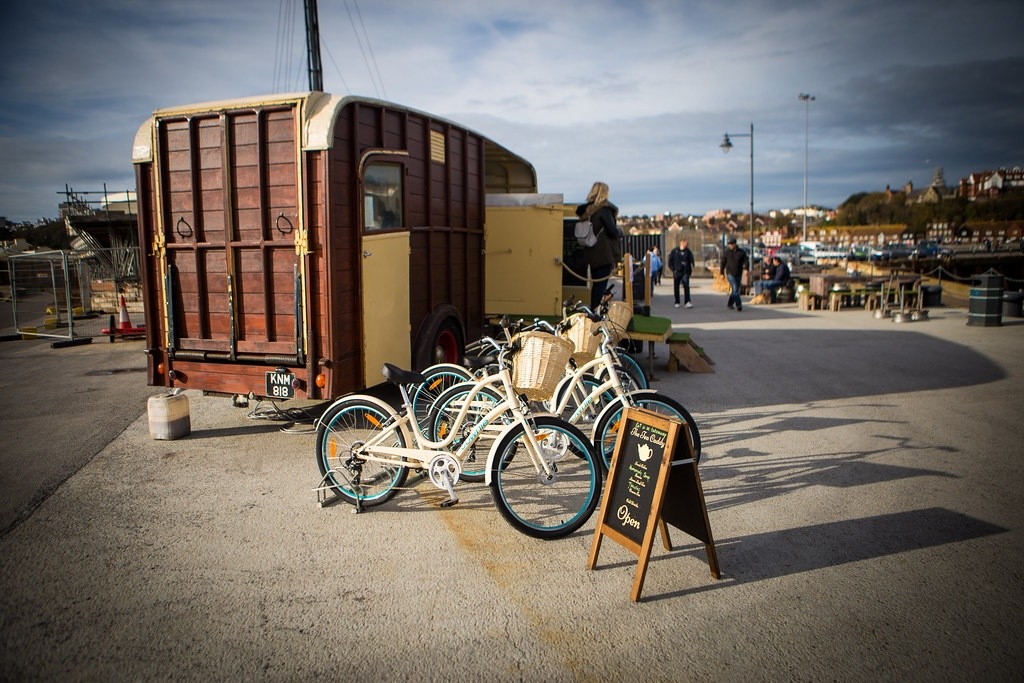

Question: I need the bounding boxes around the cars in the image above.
[768,238,959,274]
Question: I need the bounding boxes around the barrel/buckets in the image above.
[147,392,191,441]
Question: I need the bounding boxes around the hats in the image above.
[728,238,736,243]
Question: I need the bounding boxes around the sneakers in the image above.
[675,301,694,308]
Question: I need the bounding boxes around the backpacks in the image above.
[574,216,604,247]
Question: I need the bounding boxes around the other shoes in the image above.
[727,304,743,311]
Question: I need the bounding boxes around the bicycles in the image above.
[425,283,701,483]
[404,282,648,456]
[310,321,603,540]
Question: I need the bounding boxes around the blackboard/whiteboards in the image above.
[604,417,668,547]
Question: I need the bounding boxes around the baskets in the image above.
[557,313,602,368]
[510,330,576,402]
[604,301,634,342]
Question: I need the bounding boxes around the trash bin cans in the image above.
[967,275,1005,326]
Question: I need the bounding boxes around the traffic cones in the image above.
[119,295,132,329]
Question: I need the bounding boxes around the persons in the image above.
[764,256,791,304]
[643,244,663,297]
[720,237,749,312]
[753,254,777,296]
[576,182,621,316]
[667,239,695,308]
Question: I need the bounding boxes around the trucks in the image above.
[130,92,595,403]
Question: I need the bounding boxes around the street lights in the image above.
[719,122,755,270]
